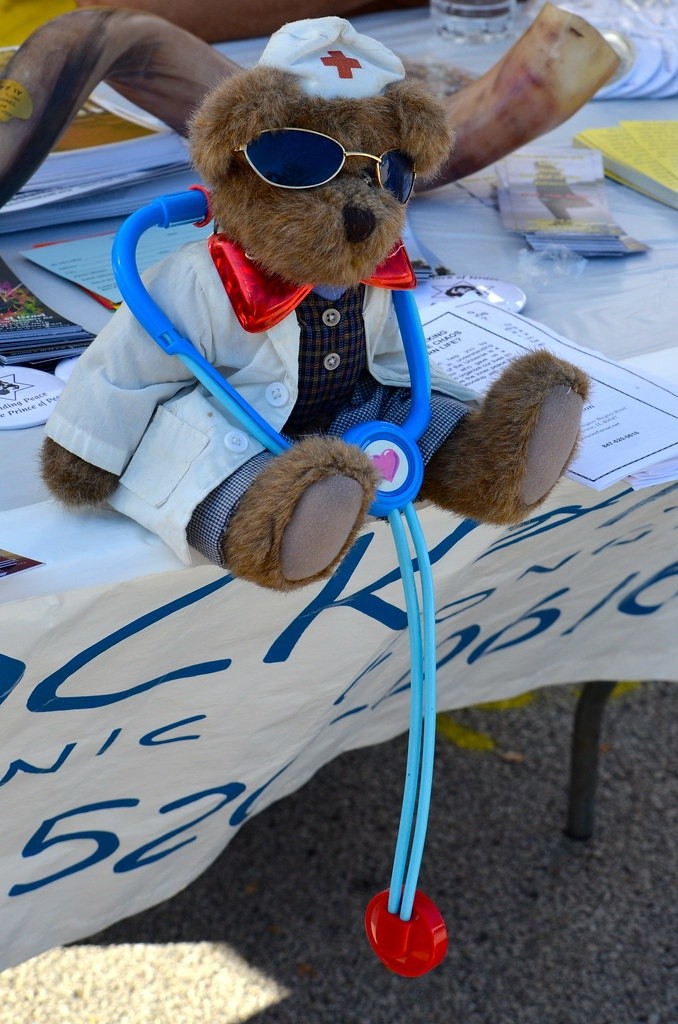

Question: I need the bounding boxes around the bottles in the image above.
[425,1,523,116]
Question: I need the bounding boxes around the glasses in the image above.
[233,126,416,206]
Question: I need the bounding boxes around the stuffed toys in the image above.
[41,15,587,590]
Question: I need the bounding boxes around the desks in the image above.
[0,0,677,976]
